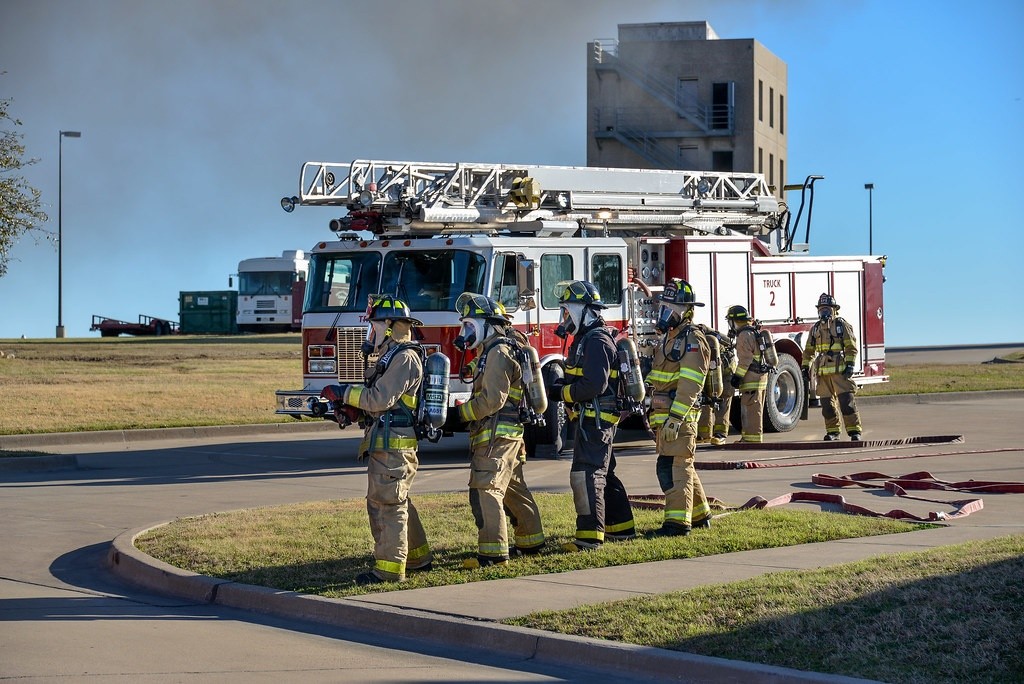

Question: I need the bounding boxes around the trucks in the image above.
[228,249,351,332]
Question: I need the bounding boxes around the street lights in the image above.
[865,183,874,254]
[56,131,82,327]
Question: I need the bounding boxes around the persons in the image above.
[695,344,736,444]
[320,294,432,585]
[645,278,712,539]
[549,280,637,552]
[438,292,545,568]
[725,305,768,443]
[801,295,862,441]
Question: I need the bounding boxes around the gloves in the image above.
[447,407,460,423]
[841,366,853,380]
[802,367,810,381]
[548,381,564,402]
[321,384,348,402]
[730,375,740,389]
[334,405,362,426]
[661,416,684,441]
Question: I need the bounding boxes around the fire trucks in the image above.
[275,158,893,448]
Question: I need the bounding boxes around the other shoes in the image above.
[508,546,540,557]
[696,436,711,443]
[824,433,839,442]
[711,434,725,445]
[561,543,586,553]
[415,562,432,572]
[357,573,384,584]
[647,526,689,538]
[691,521,710,530]
[464,556,505,568]
[851,433,861,441]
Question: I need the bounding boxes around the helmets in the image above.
[726,306,752,320]
[363,296,423,324]
[559,281,608,309]
[659,278,705,307]
[816,293,840,311]
[459,296,510,324]
